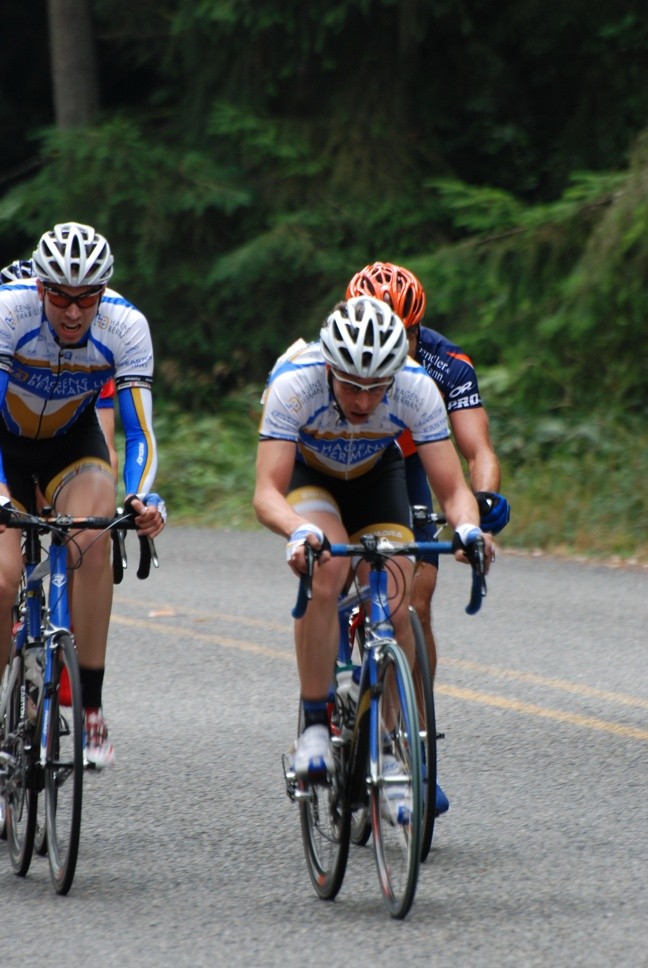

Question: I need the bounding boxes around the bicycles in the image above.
[2,497,161,893]
[279,501,492,918]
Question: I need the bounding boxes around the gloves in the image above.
[474,492,510,536]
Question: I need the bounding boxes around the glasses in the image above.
[407,325,418,341]
[329,367,393,394]
[44,284,105,309]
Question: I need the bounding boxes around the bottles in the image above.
[335,665,353,740]
[23,638,48,731]
[341,665,361,739]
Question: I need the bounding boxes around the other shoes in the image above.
[420,763,448,813]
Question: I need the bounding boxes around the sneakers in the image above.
[289,725,336,780]
[379,755,413,827]
[82,706,113,770]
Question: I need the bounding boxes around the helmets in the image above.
[345,262,427,329]
[320,295,410,378]
[31,222,114,286]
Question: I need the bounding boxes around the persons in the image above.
[345,262,511,813]
[252,300,497,826]
[0,222,168,772]
